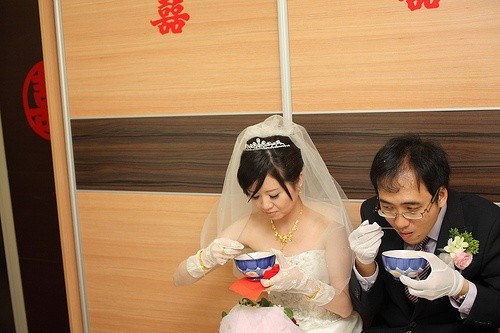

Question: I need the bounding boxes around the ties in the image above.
[404,236,431,307]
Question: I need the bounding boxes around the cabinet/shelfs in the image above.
[53,0,500,333]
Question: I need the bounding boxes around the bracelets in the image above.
[198,249,213,273]
[304,282,322,300]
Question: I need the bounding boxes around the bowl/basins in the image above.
[382,250,427,280]
[234,252,276,280]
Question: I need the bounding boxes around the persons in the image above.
[347,135,500,333]
[173,115,363,333]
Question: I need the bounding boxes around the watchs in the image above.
[452,292,468,304]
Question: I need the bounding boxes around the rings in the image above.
[268,280,274,288]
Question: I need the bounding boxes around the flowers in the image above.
[436,227,480,270]
[221,297,305,333]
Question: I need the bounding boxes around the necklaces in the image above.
[269,203,304,254]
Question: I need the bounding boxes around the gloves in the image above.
[349,219,385,265]
[186,238,245,279]
[263,248,336,306]
[400,251,464,301]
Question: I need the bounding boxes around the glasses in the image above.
[375,190,439,219]
